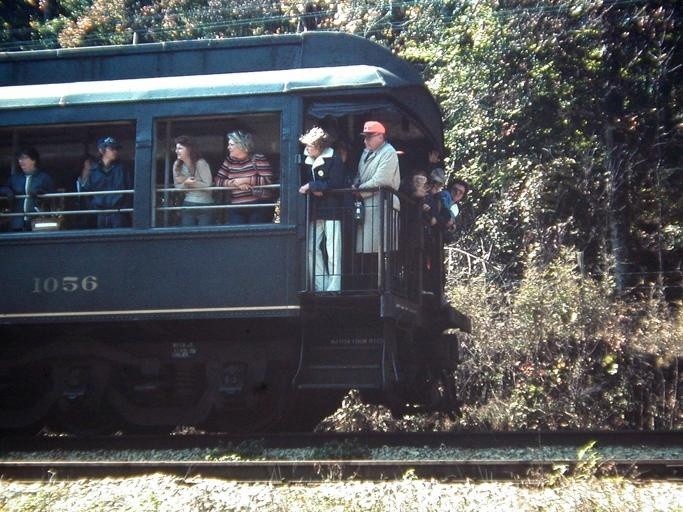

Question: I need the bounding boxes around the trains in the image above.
[0,27,473,442]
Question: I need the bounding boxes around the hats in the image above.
[431,168,445,182]
[360,121,385,136]
[95,136,123,149]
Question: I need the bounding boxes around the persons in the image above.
[173,134,216,226]
[298,127,344,295]
[82,135,134,229]
[331,120,469,294]
[75,154,96,230]
[213,129,274,224]
[0,147,56,232]
